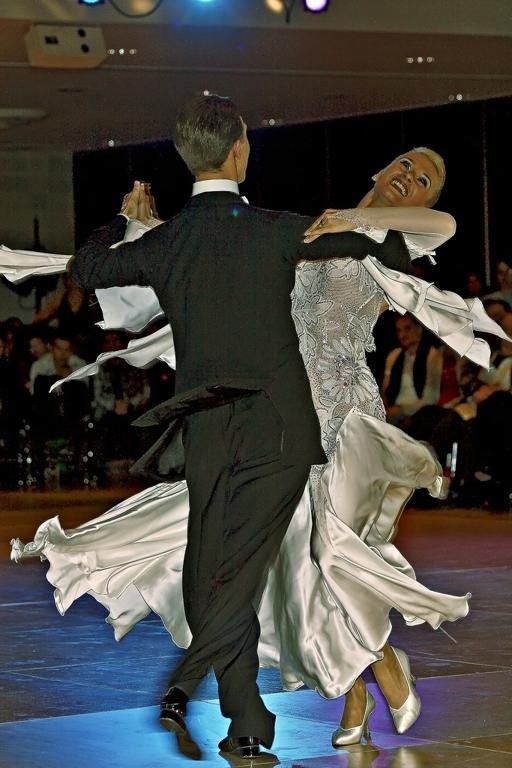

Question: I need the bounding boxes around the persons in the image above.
[1,254,511,520]
[135,135,460,746]
[341,740,426,768]
[222,748,309,768]
[65,86,414,760]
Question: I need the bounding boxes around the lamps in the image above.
[262,0,332,26]
[75,0,170,18]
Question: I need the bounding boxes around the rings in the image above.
[320,220,325,225]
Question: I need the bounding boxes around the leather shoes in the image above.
[160,691,209,761]
[219,729,264,760]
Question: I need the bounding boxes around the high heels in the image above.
[384,644,424,735]
[330,685,377,747]
